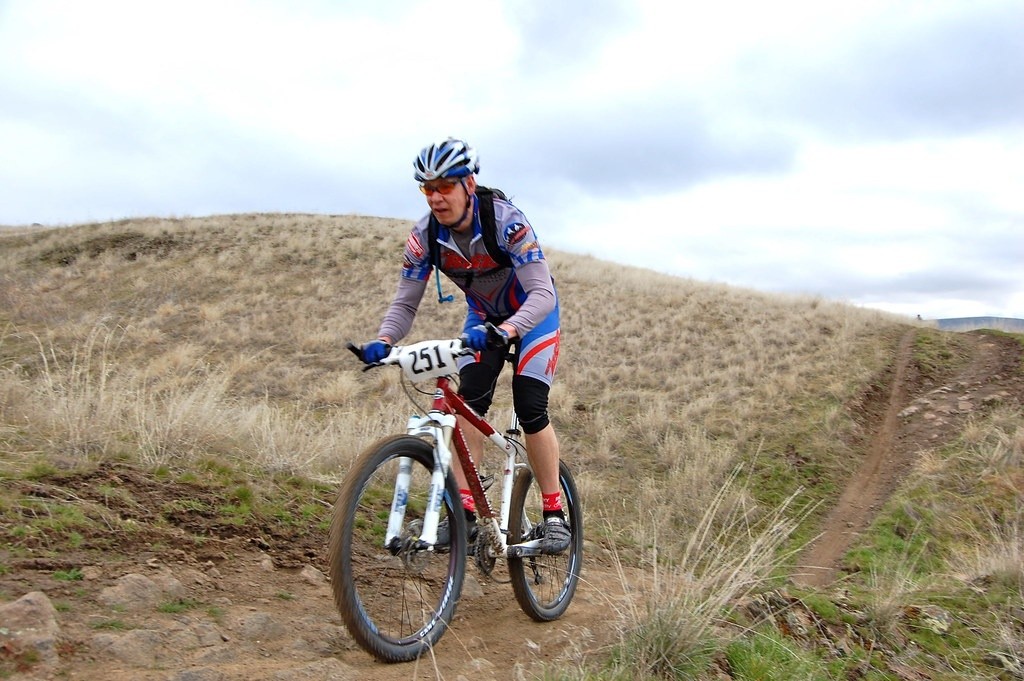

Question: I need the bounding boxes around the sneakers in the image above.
[435,512,477,548]
[541,511,571,553]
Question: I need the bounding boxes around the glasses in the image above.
[418,179,461,196]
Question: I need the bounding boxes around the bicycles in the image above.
[328,321,582,664]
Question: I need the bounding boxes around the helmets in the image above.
[413,138,480,182]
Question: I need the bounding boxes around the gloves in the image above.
[461,322,509,354]
[361,339,392,365]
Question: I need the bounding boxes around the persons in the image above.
[361,137,570,555]
